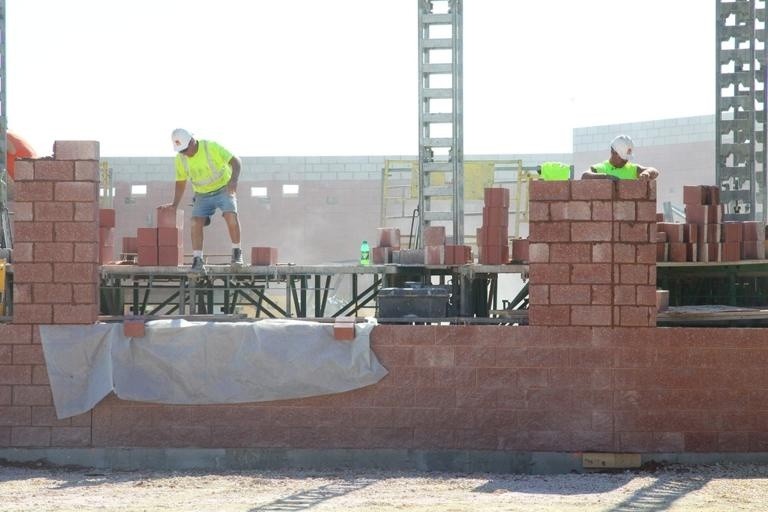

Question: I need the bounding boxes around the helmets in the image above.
[610,135,635,161]
[171,128,192,154]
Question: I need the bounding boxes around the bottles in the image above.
[360,240,370,265]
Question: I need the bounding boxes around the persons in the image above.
[580,134,659,184]
[154,128,244,269]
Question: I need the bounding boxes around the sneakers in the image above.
[192,256,206,273]
[231,246,243,266]
